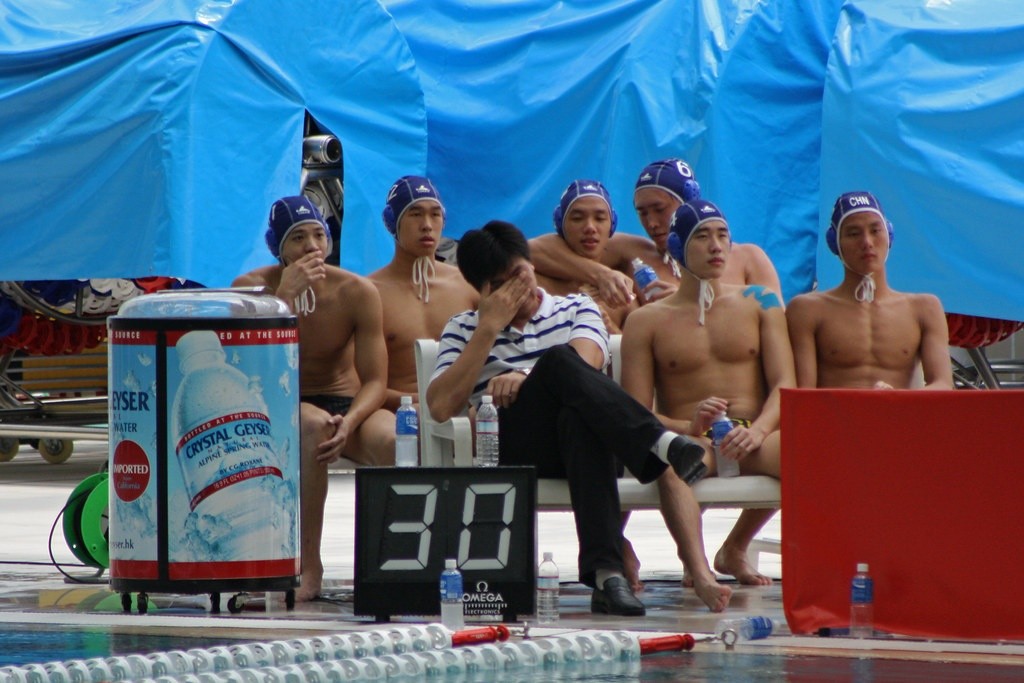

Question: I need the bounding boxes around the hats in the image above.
[266,194,331,263]
[666,198,731,268]
[826,192,894,259]
[383,177,447,239]
[634,158,700,208]
[553,179,618,240]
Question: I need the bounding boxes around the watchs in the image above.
[512,367,529,375]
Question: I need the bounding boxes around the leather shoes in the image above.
[666,436,708,486]
[590,580,646,616]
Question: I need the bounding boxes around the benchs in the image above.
[416,339,783,571]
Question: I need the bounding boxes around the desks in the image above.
[779,388,1023,644]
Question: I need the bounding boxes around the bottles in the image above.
[712,410,740,478]
[715,617,780,640]
[440,558,464,630]
[176,330,296,560]
[537,551,560,625]
[632,256,663,301]
[394,396,419,466]
[476,395,499,466]
[849,564,874,641]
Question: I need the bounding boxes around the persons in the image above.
[526,159,798,612]
[426,220,710,616]
[360,177,480,462]
[229,196,398,601]
[785,192,956,390]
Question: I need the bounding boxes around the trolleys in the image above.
[0,376,109,465]
